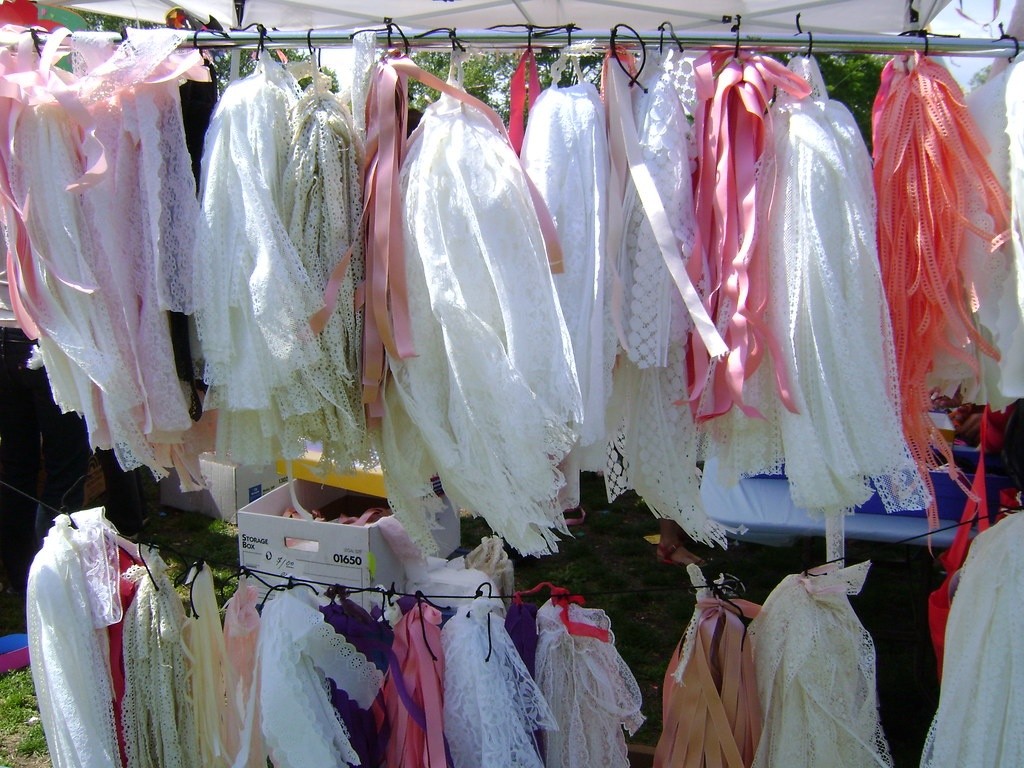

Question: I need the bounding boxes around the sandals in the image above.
[655,542,709,571]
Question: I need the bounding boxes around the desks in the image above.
[700,456,1024,714]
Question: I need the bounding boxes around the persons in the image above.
[656,518,708,568]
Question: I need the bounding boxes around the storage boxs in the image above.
[160,450,297,525]
[238,452,409,618]
